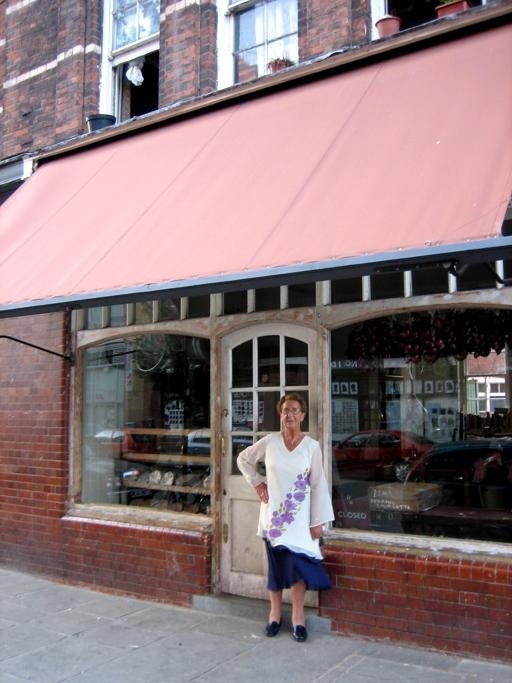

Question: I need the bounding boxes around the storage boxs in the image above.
[333,482,443,534]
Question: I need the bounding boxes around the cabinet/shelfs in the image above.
[120,428,211,515]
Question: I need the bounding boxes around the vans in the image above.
[180,425,254,456]
[399,436,512,544]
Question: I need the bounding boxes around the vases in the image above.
[86,114,116,132]
[374,17,400,39]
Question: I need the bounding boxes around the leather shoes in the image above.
[292,625,307,641]
[266,618,282,636]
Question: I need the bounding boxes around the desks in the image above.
[371,506,512,533]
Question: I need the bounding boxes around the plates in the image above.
[147,469,174,485]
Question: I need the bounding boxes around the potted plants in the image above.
[433,0,473,18]
[265,54,294,74]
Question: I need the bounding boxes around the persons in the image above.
[236,392,336,642]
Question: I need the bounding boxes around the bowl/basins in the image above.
[375,16,401,36]
[84,114,117,131]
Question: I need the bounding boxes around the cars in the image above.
[335,427,437,482]
[82,443,155,504]
